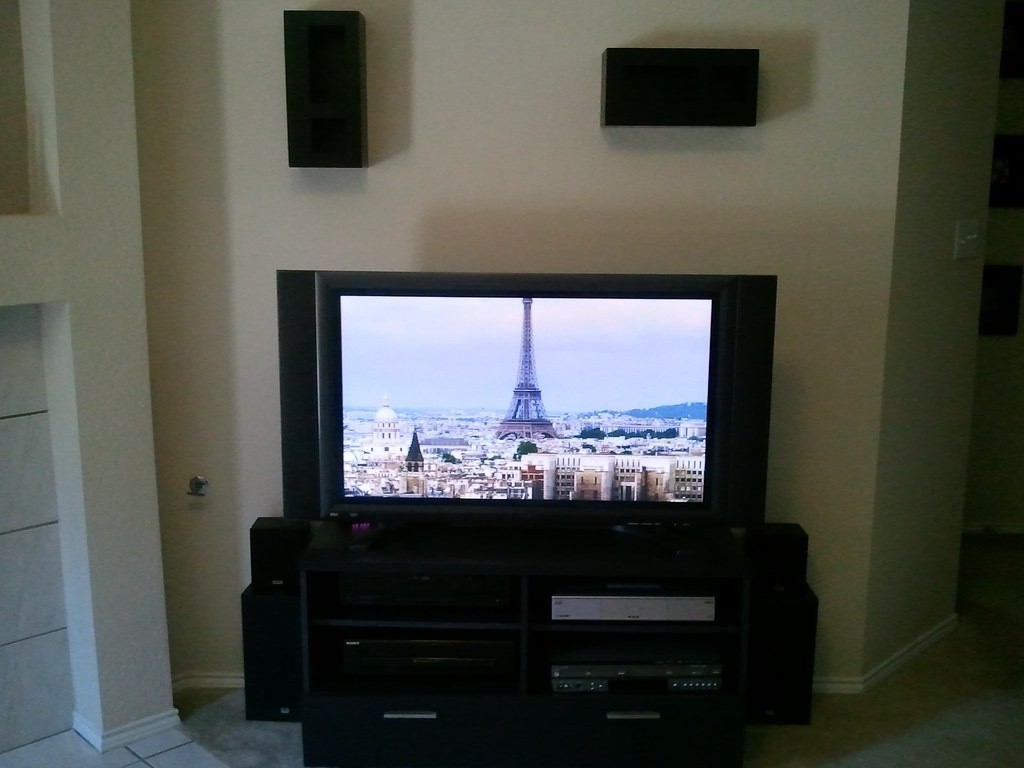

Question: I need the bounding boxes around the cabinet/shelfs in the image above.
[242,516,818,768]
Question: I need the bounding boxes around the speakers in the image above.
[250,517,312,591]
[283,11,366,171]
[242,583,303,723]
[600,49,760,129]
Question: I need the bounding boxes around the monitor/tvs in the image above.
[274,268,776,556]
